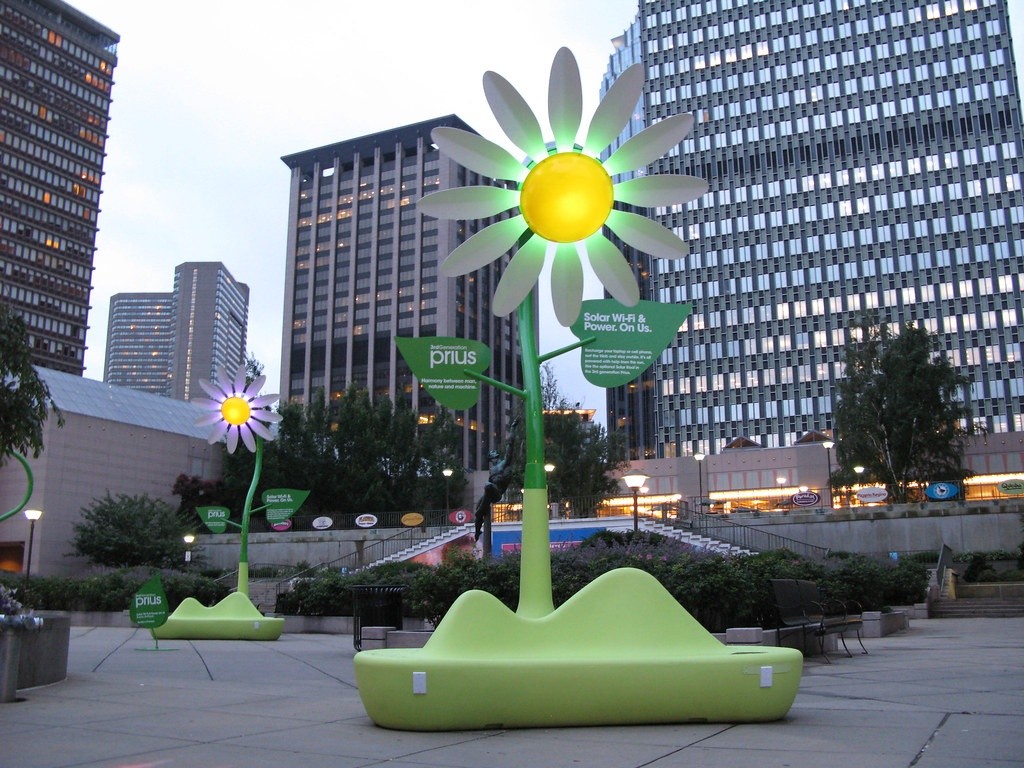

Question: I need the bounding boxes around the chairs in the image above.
[349,584,406,652]
[767,577,853,665]
[795,579,869,658]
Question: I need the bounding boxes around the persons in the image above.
[474,415,519,542]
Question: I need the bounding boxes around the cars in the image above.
[731,505,757,512]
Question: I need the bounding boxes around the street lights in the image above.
[823,440,834,508]
[854,466,865,490]
[23,509,42,590]
[694,452,706,514]
[544,462,556,510]
[442,468,453,523]
[183,533,195,576]
[619,469,649,533]
